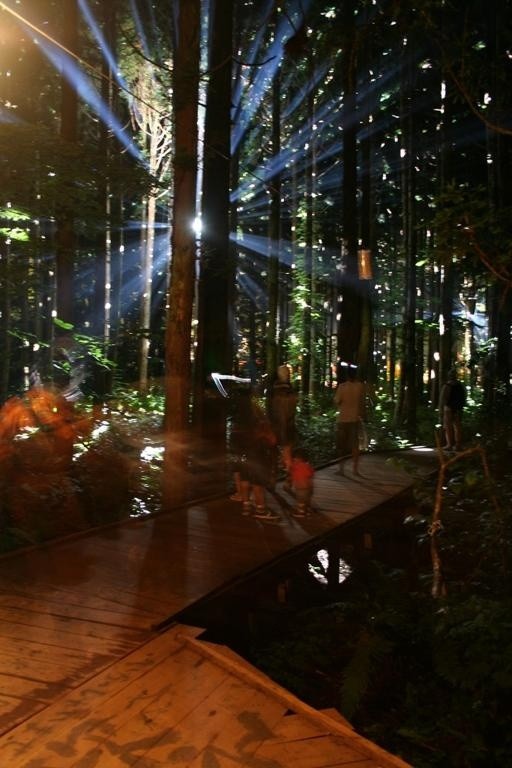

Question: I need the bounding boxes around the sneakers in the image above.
[230,480,312,519]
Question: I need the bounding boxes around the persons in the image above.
[220,359,317,522]
[439,368,466,451]
[331,368,365,478]
